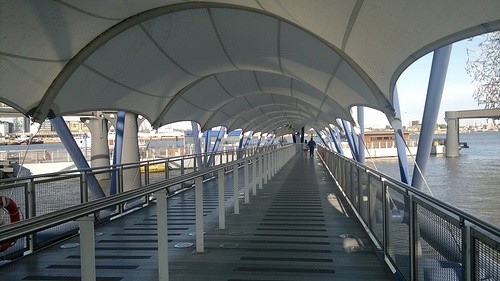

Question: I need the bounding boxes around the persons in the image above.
[308,138,316,156]
[303,139,309,157]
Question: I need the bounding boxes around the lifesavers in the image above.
[0,196,20,253]
